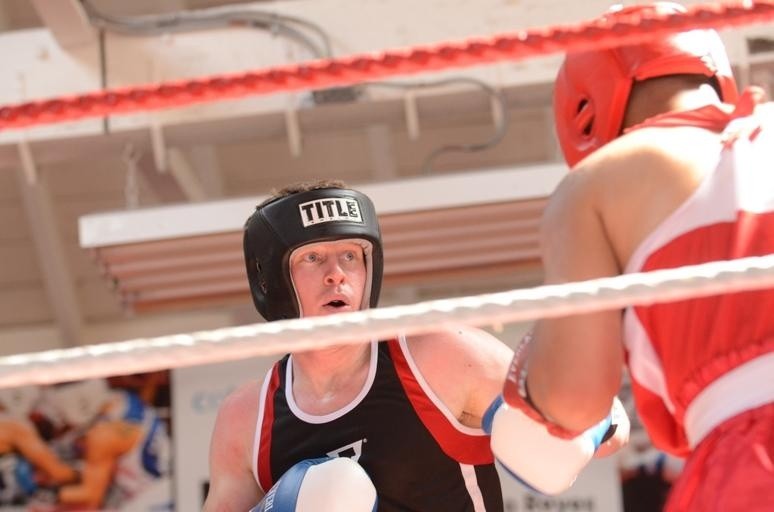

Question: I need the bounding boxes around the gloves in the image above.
[481,327,618,496]
[247,457,379,512]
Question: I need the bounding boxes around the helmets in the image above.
[551,2,741,169]
[242,187,382,322]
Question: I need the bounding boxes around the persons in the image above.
[198,180,633,512]
[0,379,176,509]
[479,1,773,512]
